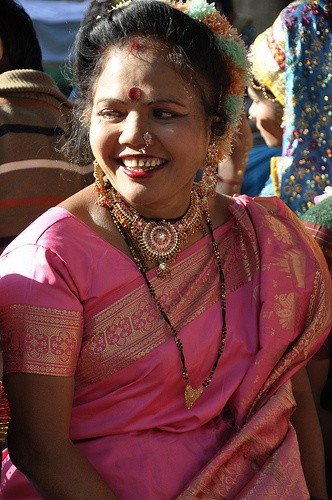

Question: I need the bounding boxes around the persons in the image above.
[0,2,96,253]
[0,1,330,500]
[214,0,331,500]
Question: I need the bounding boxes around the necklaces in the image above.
[106,187,228,408]
[98,174,208,277]
